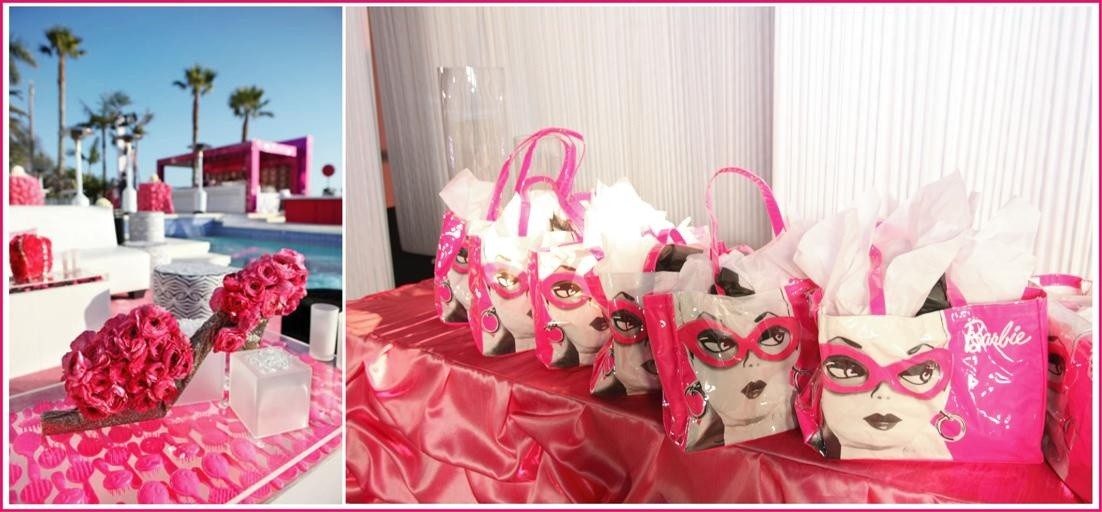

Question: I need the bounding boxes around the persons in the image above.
[444,236,958,459]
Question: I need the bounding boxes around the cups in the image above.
[309,302,340,361]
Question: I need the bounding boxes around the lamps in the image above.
[153,263,231,406]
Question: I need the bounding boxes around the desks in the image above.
[345,277,1086,504]
[9,329,343,505]
[8,270,110,381]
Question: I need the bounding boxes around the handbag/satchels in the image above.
[10,176,44,206]
[136,181,174,214]
[794,221,1048,462]
[10,235,53,283]
[434,128,809,453]
[1030,273,1092,499]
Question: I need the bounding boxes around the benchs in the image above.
[8,204,150,298]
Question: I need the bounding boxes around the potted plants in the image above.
[40,248,309,436]
